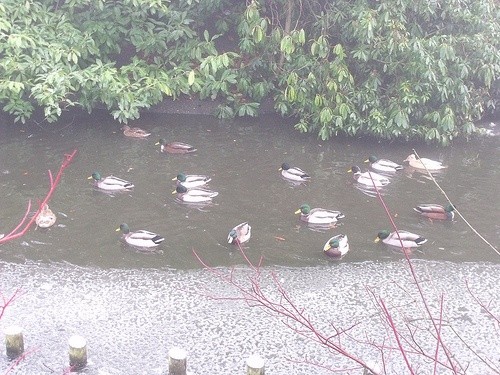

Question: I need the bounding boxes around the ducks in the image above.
[120,125,151,137]
[365,156,404,172]
[156,138,198,153]
[227,221,251,243]
[413,202,457,220]
[294,204,345,223]
[172,174,212,188]
[347,165,391,186]
[375,230,428,248]
[172,185,218,201]
[279,163,311,181]
[404,154,445,170]
[88,172,134,189]
[116,224,164,246]
[36,205,56,228]
[323,234,349,257]
